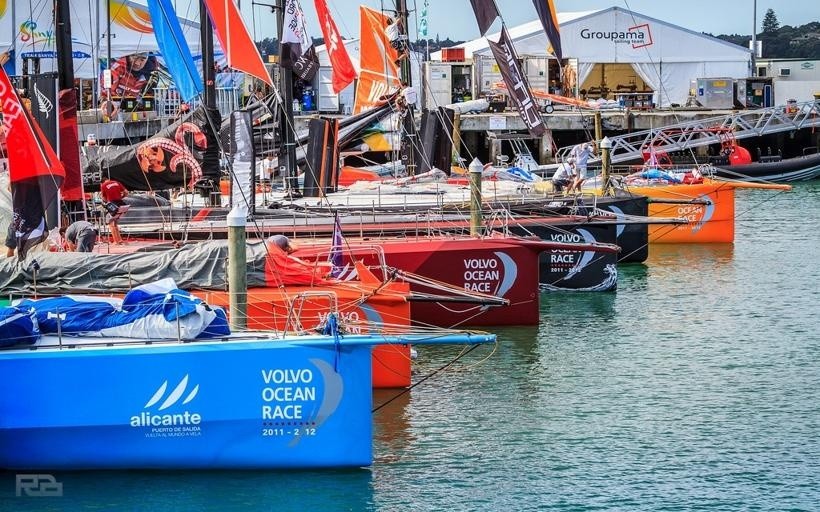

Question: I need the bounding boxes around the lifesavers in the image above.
[101,100,118,122]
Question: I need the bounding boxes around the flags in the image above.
[202,0,275,88]
[147,0,206,106]
[312,0,357,95]
[531,0,564,63]
[470,0,499,37]
[279,0,321,84]
[78,105,222,192]
[484,23,547,139]
[328,204,343,280]
[353,4,402,114]
[0,63,65,262]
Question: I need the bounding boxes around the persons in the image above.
[394,82,418,117]
[259,153,275,188]
[103,51,159,97]
[383,17,410,68]
[214,59,242,73]
[5,219,18,259]
[59,219,98,254]
[181,101,190,113]
[100,176,130,246]
[570,139,598,192]
[552,157,575,195]
[255,87,263,98]
